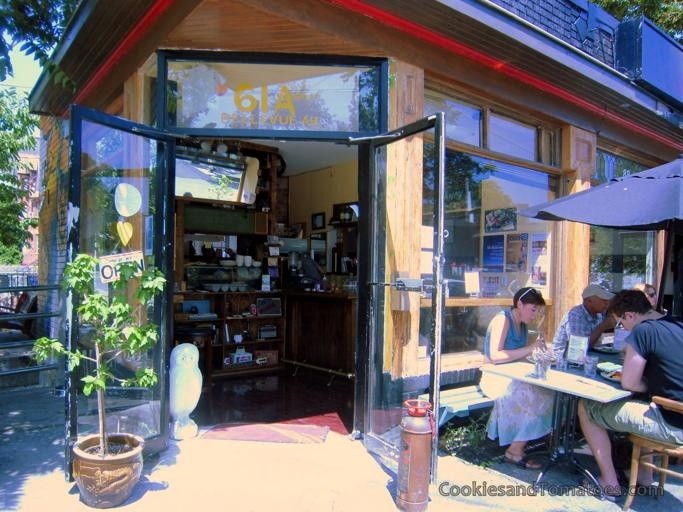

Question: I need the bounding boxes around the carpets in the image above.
[199,423,331,445]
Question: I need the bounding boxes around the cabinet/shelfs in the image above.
[173,289,290,380]
[326,221,359,275]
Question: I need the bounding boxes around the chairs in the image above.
[622,395,683,512]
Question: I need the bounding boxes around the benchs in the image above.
[0,292,37,340]
[417,384,494,429]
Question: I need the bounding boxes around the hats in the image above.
[581,283,616,301]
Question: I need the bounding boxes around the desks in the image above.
[287,289,358,383]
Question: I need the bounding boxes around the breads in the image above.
[596,361,624,378]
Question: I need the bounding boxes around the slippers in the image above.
[615,469,653,497]
[502,454,543,470]
[574,475,624,503]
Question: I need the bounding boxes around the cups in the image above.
[583,355,597,378]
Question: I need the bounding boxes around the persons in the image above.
[477,285,556,470]
[574,288,681,503]
[633,282,658,309]
[551,282,620,352]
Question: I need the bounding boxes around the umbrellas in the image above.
[509,152,683,230]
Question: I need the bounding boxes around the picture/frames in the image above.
[311,212,325,230]
[181,299,212,314]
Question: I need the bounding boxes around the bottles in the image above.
[532,331,547,378]
[338,201,353,222]
[287,250,299,272]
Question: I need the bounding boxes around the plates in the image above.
[599,364,622,382]
[218,254,263,267]
[525,352,560,367]
[592,344,621,355]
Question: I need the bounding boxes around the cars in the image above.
[0,271,38,337]
[420,278,475,350]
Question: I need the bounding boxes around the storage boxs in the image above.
[253,350,278,365]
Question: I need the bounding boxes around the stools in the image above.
[174,321,216,427]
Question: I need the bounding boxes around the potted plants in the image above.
[32,254,167,509]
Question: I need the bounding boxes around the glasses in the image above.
[614,313,625,329]
[647,292,656,298]
[519,286,544,303]
